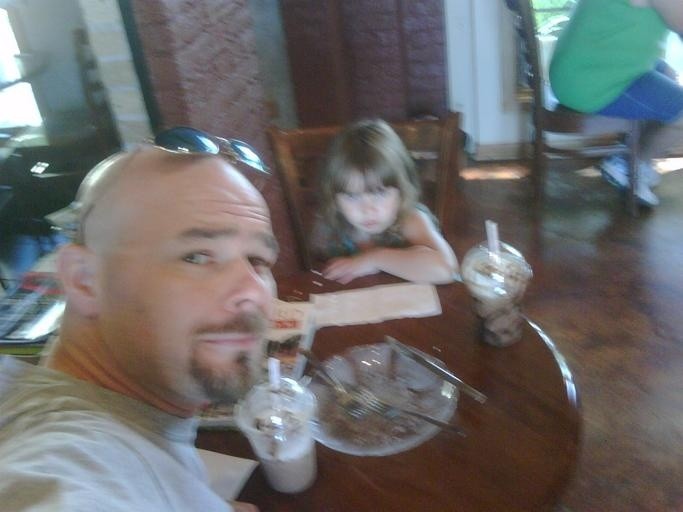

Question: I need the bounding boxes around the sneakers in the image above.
[591,150,669,211]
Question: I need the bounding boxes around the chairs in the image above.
[266,111,461,267]
[519,0,640,213]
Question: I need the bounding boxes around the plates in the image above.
[300,346,458,459]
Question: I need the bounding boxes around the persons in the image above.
[0,141,283,512]
[300,118,461,287]
[547,1,683,210]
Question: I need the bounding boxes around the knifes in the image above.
[382,333,489,407]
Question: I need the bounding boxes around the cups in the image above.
[459,245,533,348]
[231,379,320,494]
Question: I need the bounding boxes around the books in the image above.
[194,298,324,432]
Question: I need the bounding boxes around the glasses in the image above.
[73,124,275,247]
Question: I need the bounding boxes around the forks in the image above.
[335,381,466,450]
